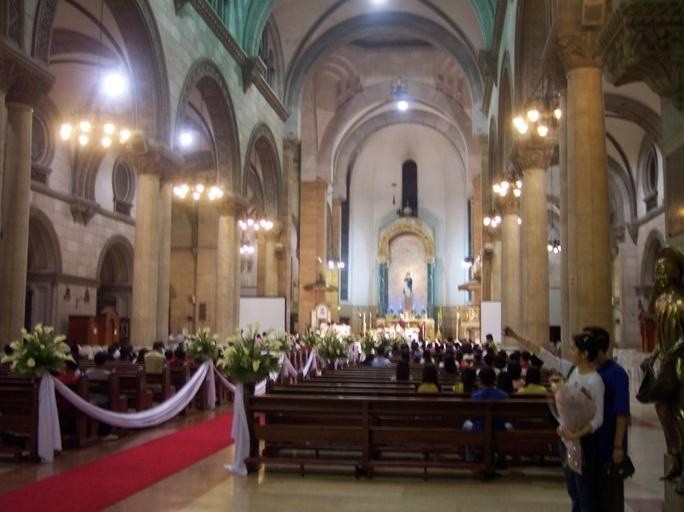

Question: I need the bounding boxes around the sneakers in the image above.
[102,433,118,441]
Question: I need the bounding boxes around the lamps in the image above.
[547,159,561,254]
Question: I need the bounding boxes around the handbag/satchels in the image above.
[603,456,635,480]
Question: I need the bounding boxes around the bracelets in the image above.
[613,444,623,450]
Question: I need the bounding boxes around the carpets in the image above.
[0,410,236,512]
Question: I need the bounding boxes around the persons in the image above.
[582,324,633,512]
[635,254,684,495]
[368,329,550,481]
[0,335,198,445]
[500,324,606,512]
[401,271,415,311]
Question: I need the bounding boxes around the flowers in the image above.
[1,320,77,378]
[182,320,378,386]
